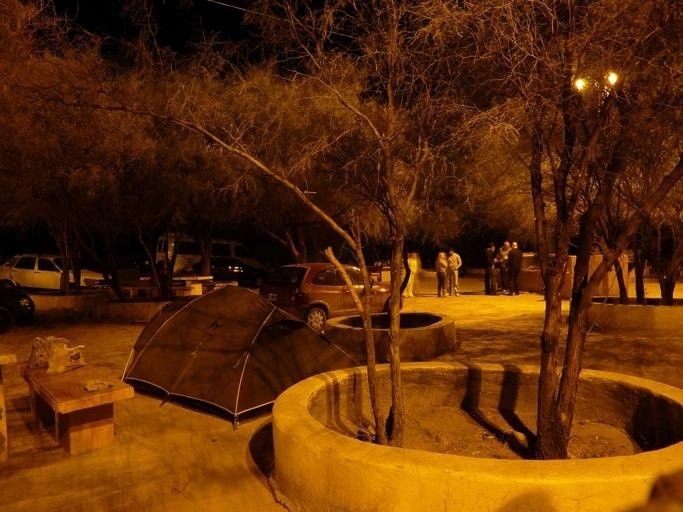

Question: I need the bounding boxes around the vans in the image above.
[152,233,259,277]
[260,260,403,335]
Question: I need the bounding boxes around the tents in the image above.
[123,285,362,431]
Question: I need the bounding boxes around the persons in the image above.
[435,252,449,298]
[401,253,419,298]
[484,243,499,296]
[506,242,523,295]
[496,241,512,293]
[448,249,463,296]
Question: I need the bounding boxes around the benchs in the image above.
[121,286,193,296]
[207,281,239,294]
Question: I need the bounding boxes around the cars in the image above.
[0,276,37,332]
[173,256,276,292]
[0,251,115,294]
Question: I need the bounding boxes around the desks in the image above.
[16,352,135,456]
[172,276,213,296]
